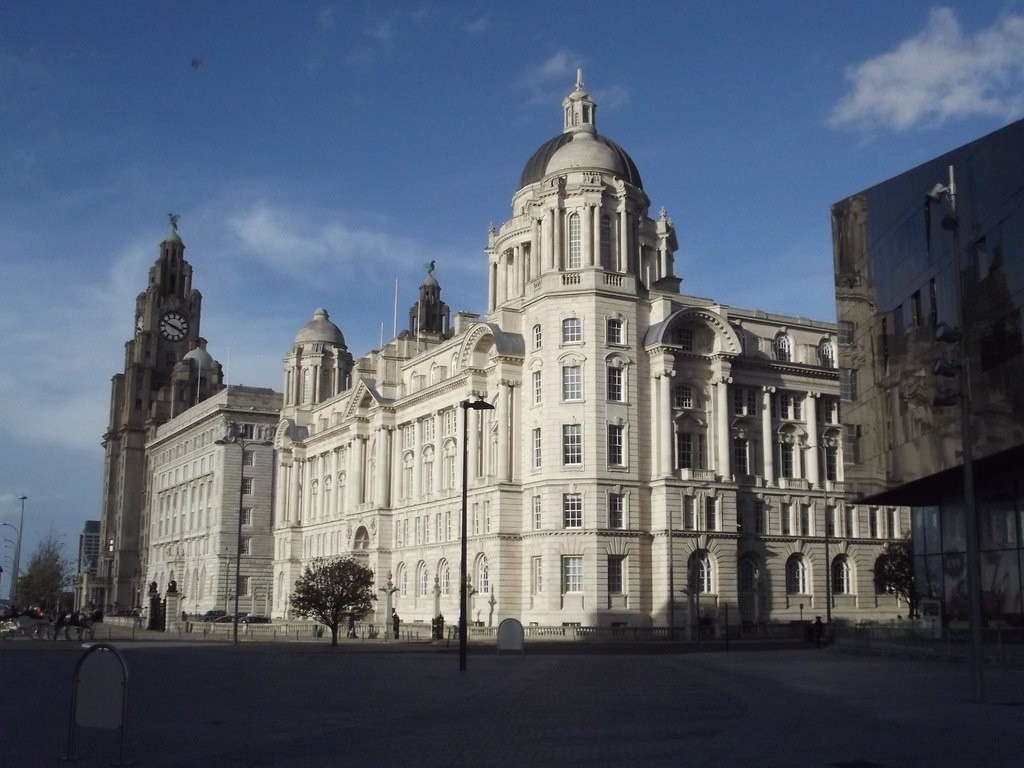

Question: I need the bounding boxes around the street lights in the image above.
[454,400,497,669]
[799,444,836,644]
[213,438,276,643]
[0,494,28,606]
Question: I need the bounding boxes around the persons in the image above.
[700,613,714,634]
[392,613,400,639]
[346,612,358,638]
[812,616,824,649]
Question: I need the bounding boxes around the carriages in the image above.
[31,609,105,643]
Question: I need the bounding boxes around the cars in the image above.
[0,618,24,638]
